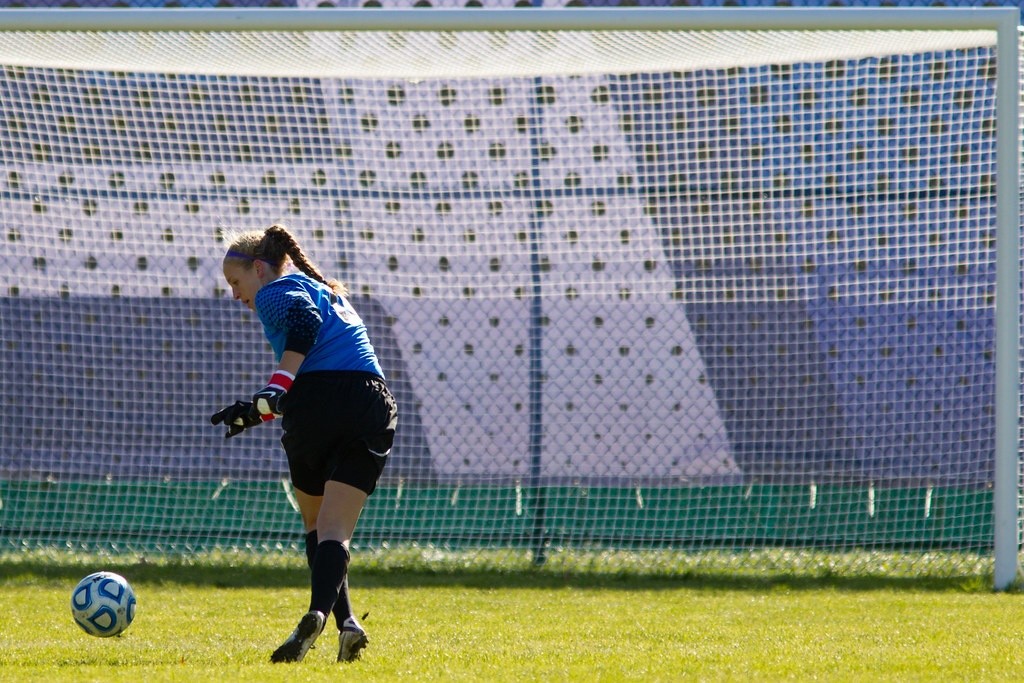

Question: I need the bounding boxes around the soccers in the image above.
[69,571,137,639]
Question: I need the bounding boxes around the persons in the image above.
[210,226,399,665]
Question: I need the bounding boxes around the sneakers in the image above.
[337,615,369,663]
[271,610,325,664]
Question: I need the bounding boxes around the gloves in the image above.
[211,400,283,439]
[249,369,296,423]
